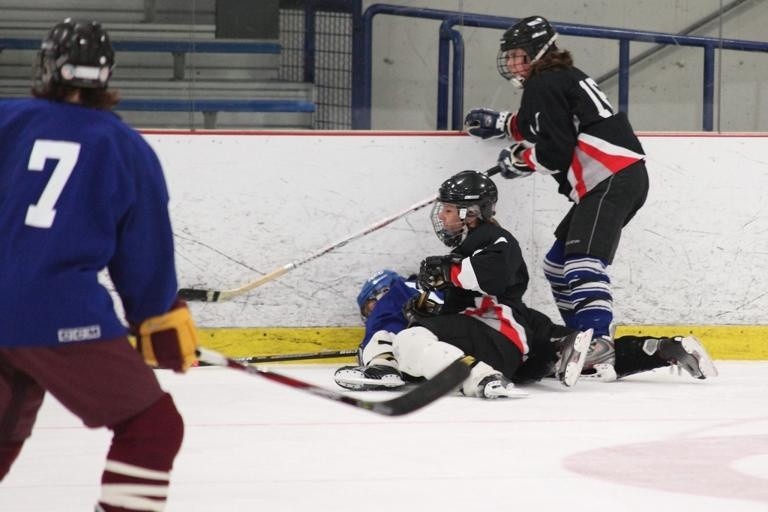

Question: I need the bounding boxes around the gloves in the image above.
[497,142,539,180]
[418,252,463,294]
[401,296,444,328]
[134,299,202,375]
[464,107,515,140]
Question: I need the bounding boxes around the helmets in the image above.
[430,169,498,248]
[32,16,117,91]
[496,15,560,90]
[357,269,407,323]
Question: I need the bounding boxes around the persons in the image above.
[467,14,649,386]
[0,12,187,512]
[334,167,532,400]
[356,268,718,389]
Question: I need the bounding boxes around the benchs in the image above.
[0,28,285,82]
[113,96,318,130]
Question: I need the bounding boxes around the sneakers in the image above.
[554,330,583,381]
[552,322,615,371]
[656,336,705,383]
[479,375,515,399]
[333,359,407,393]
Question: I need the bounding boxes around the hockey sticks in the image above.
[177,167,501,301]
[195,350,470,416]
